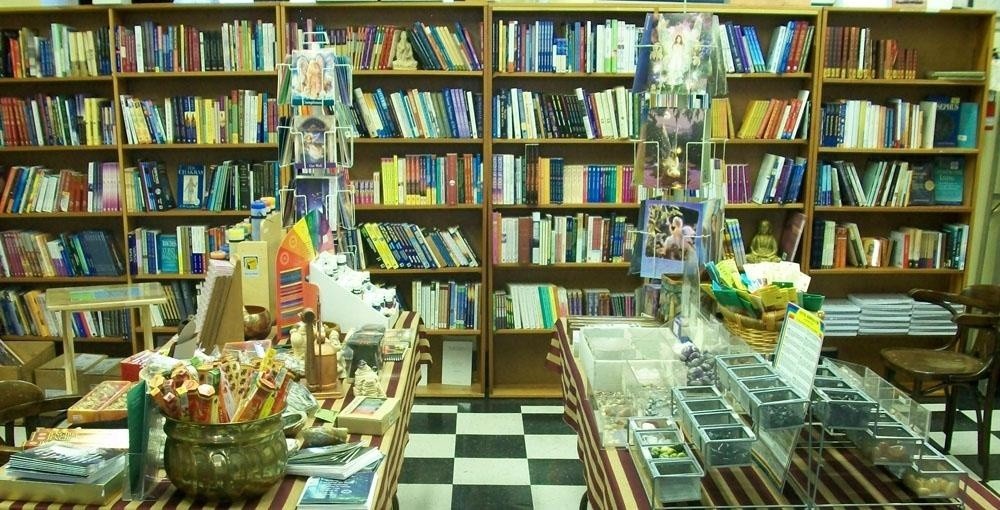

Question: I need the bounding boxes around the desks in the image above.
[1,310,422,510]
[558,316,1000,509]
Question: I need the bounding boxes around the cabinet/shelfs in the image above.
[3,3,995,401]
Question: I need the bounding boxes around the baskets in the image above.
[716,302,825,361]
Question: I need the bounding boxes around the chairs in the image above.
[1,380,83,466]
[879,285,1000,482]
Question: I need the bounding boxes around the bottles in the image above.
[250,201,267,242]
